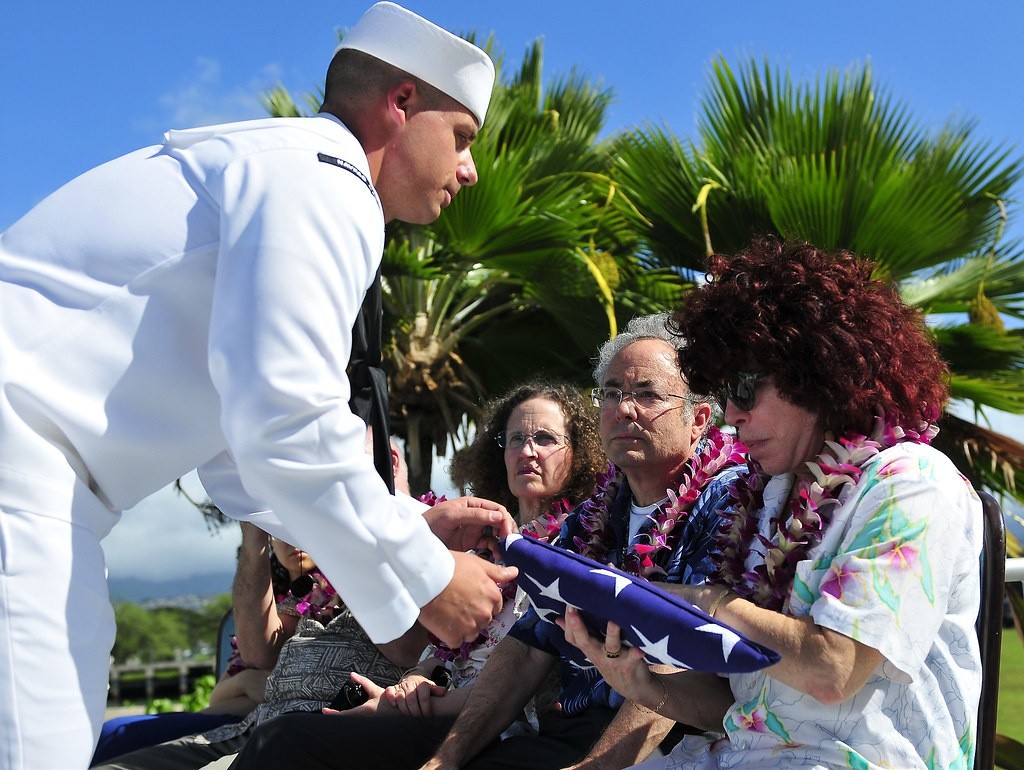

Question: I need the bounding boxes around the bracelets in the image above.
[629,670,669,714]
[709,590,746,618]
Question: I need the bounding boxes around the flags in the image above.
[499,533,781,674]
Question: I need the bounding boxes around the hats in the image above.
[331,1,496,131]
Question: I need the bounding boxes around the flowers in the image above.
[225,420,941,663]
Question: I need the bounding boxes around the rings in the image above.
[603,646,624,658]
[486,526,493,537]
[395,690,405,695]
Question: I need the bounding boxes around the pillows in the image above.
[497,533,782,672]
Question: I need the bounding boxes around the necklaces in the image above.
[296,490,448,615]
[426,493,575,662]
[226,586,293,678]
[573,426,751,580]
[706,414,940,613]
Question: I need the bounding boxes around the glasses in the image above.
[588,385,699,409]
[494,429,571,449]
[711,371,774,414]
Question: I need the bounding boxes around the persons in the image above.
[88,533,347,770]
[0,0,520,770]
[417,314,752,770]
[89,436,410,770]
[555,234,983,769]
[228,382,611,770]
